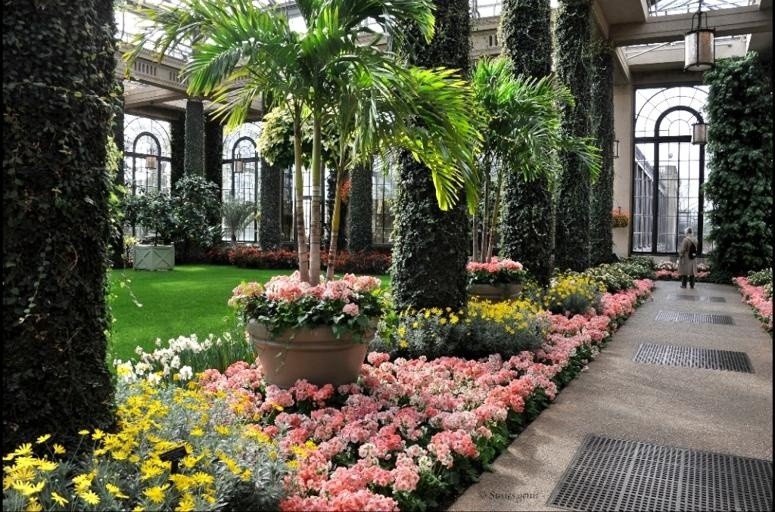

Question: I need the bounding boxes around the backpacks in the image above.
[687,237,697,259]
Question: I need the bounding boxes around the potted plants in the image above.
[123,0,478,392]
[467,55,604,304]
[132,173,222,271]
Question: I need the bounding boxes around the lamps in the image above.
[683,0,716,72]
[612,139,620,159]
[233,153,244,174]
[691,111,709,145]
[145,148,156,169]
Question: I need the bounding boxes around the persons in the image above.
[678,227,697,288]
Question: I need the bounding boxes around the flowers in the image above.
[467,257,523,284]
[610,208,630,219]
[226,270,382,320]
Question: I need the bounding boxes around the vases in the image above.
[466,283,522,302]
[611,216,628,228]
